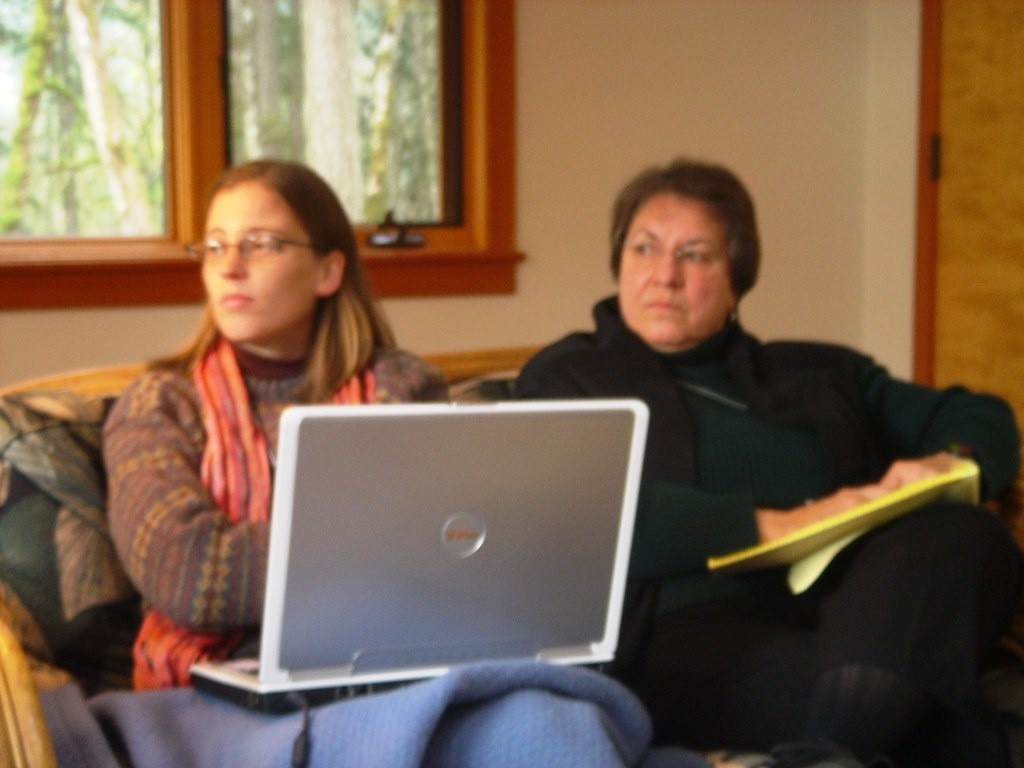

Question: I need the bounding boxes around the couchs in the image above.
[1,341,865,768]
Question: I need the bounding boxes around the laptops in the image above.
[188,397,652,719]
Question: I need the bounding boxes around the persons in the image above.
[98,158,658,768]
[511,156,1021,767]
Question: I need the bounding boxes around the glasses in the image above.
[183,232,320,261]
